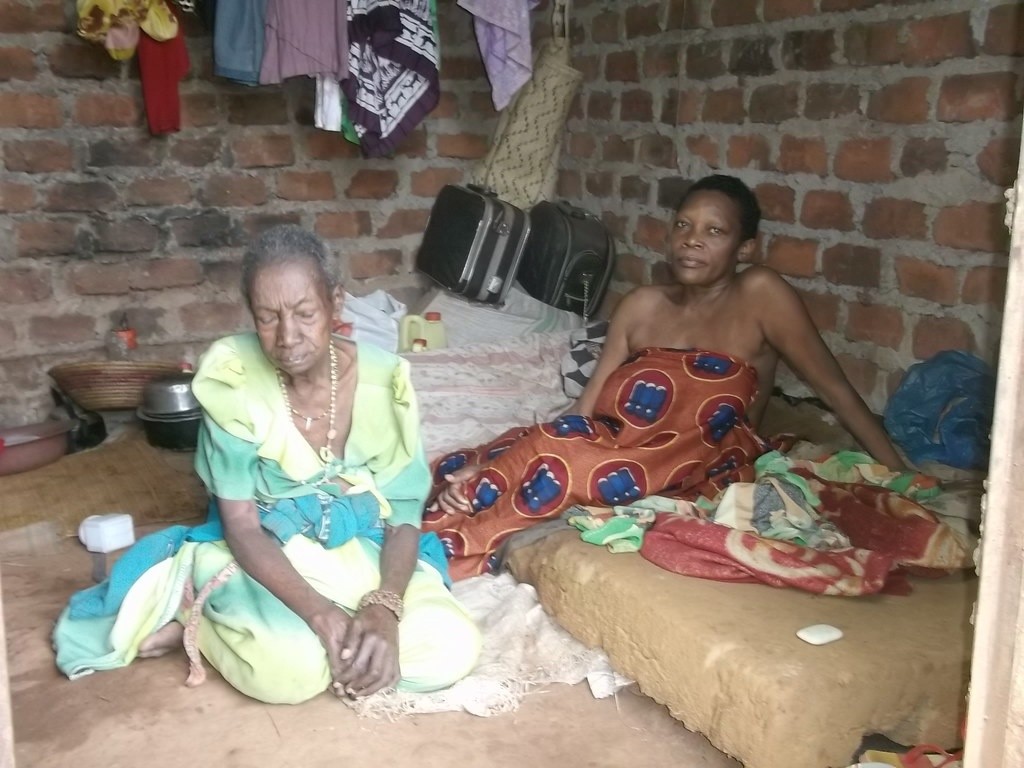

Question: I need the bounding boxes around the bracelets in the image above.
[358,589,406,624]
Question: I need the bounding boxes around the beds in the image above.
[499,389,986,767]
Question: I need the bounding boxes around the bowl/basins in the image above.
[137,372,202,453]
[0,421,76,475]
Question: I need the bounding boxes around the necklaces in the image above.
[271,340,339,488]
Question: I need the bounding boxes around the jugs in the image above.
[398,309,446,352]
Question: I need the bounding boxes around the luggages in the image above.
[517,200,616,318]
[417,182,532,310]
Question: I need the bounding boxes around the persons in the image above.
[135,223,485,706]
[418,174,919,586]
